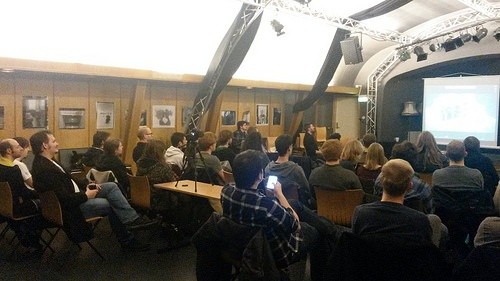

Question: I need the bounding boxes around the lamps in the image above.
[411,45,428,62]
[397,47,411,62]
[493,27,500,41]
[402,102,419,142]
[429,26,488,52]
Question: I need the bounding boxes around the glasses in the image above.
[145,131,154,136]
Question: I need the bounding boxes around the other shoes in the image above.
[161,221,178,233]
[24,230,44,249]
[9,221,29,249]
[452,245,475,267]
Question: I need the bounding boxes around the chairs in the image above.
[298,125,327,149]
[24,182,108,262]
[267,136,278,154]
[313,186,364,226]
[0,181,56,259]
[82,164,155,210]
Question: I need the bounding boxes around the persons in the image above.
[84,131,133,198]
[29,130,158,250]
[0,137,43,248]
[132,121,500,281]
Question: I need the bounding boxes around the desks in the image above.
[153,180,224,200]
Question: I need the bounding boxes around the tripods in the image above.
[175,141,214,192]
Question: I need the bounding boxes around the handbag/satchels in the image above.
[71,150,84,169]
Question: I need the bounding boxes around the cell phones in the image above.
[89,184,96,190]
[266,175,278,189]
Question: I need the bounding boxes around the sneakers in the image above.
[125,212,160,230]
[120,237,152,252]
